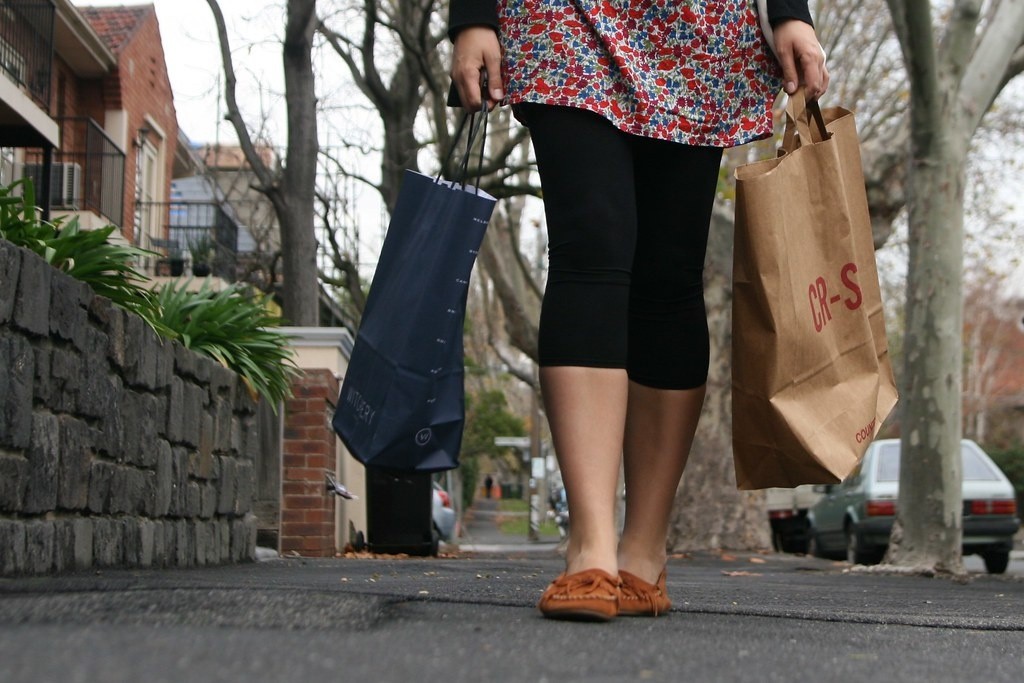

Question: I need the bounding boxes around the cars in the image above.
[432,481,455,539]
[803,437,1019,574]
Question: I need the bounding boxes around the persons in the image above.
[484,474,493,498]
[445,0,829,619]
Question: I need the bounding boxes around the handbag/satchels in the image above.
[332,97,497,473]
[729,87,899,492]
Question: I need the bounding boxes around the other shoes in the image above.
[618,565,671,615]
[539,567,622,621]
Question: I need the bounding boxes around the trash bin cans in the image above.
[355,467,439,558]
